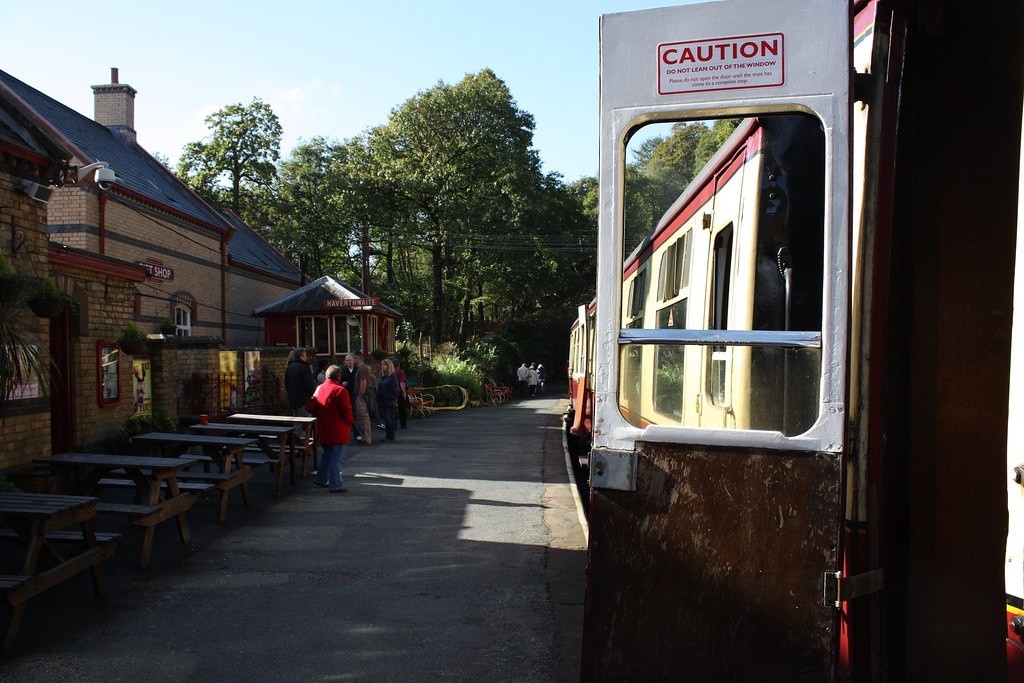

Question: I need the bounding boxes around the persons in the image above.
[304,364,356,492]
[518,362,553,397]
[284,346,407,444]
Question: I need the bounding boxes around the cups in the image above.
[200,414,208,425]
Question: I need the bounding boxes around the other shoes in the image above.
[401,426,408,429]
[356,439,372,446]
[313,479,329,488]
[356,436,362,440]
[530,394,535,400]
[329,488,348,493]
[381,438,393,442]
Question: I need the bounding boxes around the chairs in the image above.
[485,378,514,405]
[406,390,434,417]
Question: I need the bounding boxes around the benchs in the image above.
[0,435,314,606]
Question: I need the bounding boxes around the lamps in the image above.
[22,179,54,203]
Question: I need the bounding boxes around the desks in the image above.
[190,423,295,496]
[133,432,249,523]
[32,451,199,552]
[0,493,98,645]
[227,414,317,478]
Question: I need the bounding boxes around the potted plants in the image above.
[26,273,80,318]
[160,318,176,335]
[0,255,23,302]
[656,363,684,418]
[400,343,411,357]
[372,344,387,361]
[118,321,145,354]
